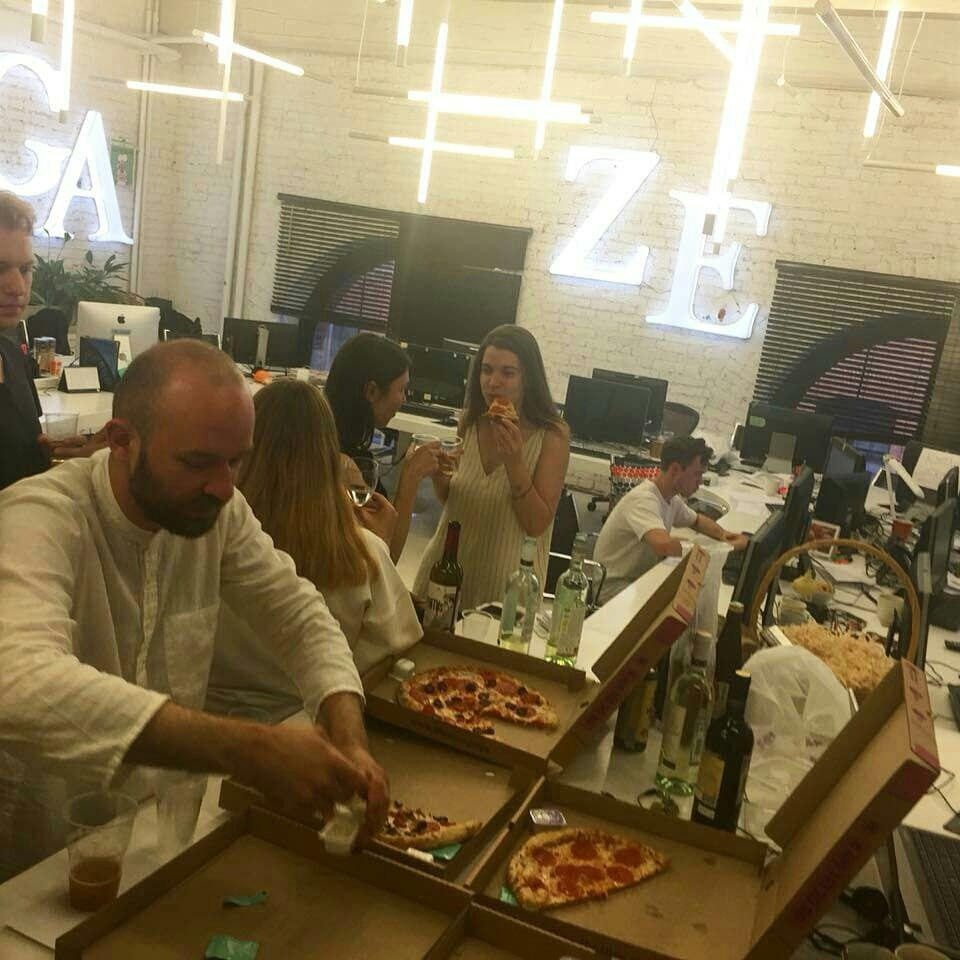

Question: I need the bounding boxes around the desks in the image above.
[37,384,113,436]
[388,406,644,499]
[1,463,960,960]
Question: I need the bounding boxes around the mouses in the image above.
[440,415,457,425]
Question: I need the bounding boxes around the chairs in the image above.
[543,488,608,617]
[587,401,700,524]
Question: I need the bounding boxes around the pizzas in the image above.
[396,666,559,738]
[509,828,668,912]
[487,398,520,426]
[381,798,478,848]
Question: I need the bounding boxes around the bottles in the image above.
[654,630,712,797]
[423,520,464,634]
[666,612,710,703]
[614,669,656,753]
[33,337,57,360]
[705,602,744,721]
[691,669,755,836]
[498,537,539,655]
[544,532,589,669]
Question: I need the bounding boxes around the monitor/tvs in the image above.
[725,402,872,625]
[75,301,160,371]
[400,343,471,410]
[885,467,960,676]
[563,368,669,448]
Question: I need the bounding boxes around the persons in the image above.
[0,336,392,884]
[322,330,451,636]
[410,323,571,635]
[0,189,57,493]
[589,435,751,608]
[234,377,426,680]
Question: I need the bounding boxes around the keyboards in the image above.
[723,549,746,571]
[398,403,454,419]
[765,503,785,514]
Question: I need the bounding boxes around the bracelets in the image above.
[512,480,534,501]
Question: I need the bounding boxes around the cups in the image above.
[878,594,904,628]
[765,476,780,497]
[150,764,207,862]
[438,436,464,476]
[61,792,140,912]
[344,457,378,510]
[412,434,440,452]
[462,609,493,642]
[44,412,78,463]
[38,351,64,377]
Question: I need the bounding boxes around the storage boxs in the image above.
[56,544,941,960]
